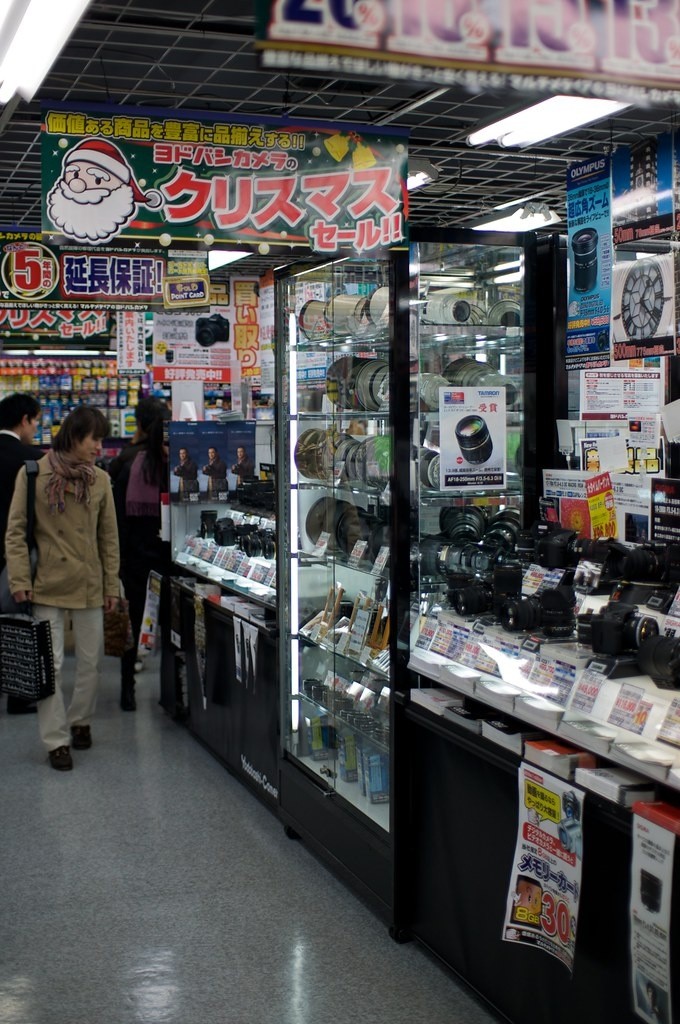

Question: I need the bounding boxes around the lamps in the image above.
[449,92,634,150]
[454,154,562,233]
[407,156,443,190]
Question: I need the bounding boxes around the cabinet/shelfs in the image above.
[273,222,538,924]
[157,575,281,819]
[390,689,680,1024]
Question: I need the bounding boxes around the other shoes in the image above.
[70,725,92,749]
[7,695,37,715]
[134,659,144,672]
[48,745,72,771]
[120,688,136,711]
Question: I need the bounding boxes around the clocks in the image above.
[613,258,673,341]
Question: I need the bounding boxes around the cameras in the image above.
[195,314,230,346]
[449,522,680,689]
[216,517,277,560]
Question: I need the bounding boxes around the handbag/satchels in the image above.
[0,600,55,701]
[0,536,39,617]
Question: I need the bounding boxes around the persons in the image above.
[0,392,172,771]
[174,445,255,503]
